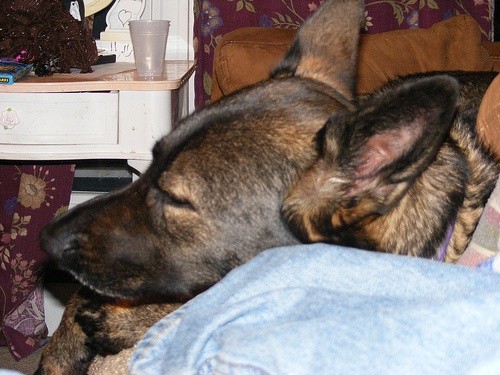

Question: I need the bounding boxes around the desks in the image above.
[0,58,198,184]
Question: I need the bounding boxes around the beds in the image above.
[127,0,500,375]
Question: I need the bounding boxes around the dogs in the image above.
[33,0,500,375]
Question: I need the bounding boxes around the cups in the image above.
[129,20,170,76]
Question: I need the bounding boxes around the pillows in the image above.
[210,13,485,106]
